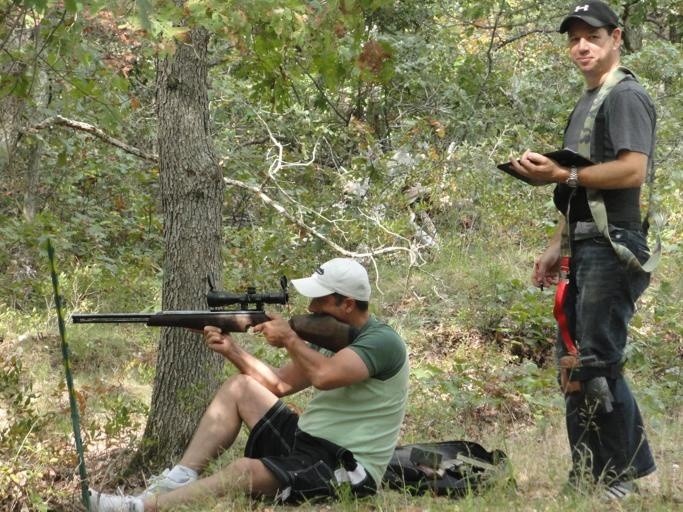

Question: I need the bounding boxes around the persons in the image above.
[85,256,408,511]
[504,2,658,511]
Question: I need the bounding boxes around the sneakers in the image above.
[562,470,660,505]
[88,468,198,512]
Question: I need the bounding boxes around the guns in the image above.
[72,270,358,352]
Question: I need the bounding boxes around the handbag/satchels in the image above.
[381,441,517,499]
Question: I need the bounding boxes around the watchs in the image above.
[567,166,580,191]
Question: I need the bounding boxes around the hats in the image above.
[290,258,371,301]
[560,0,618,34]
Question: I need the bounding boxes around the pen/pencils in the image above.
[535,263,543,292]
